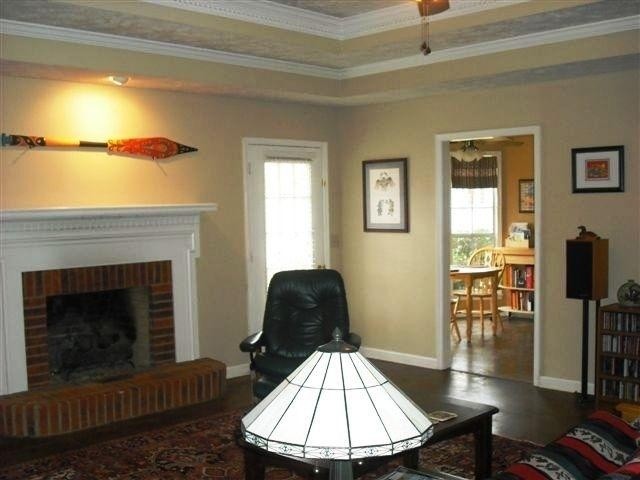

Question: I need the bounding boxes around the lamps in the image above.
[235,324,437,480]
[447,138,486,163]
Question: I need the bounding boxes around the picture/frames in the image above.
[568,142,627,194]
[516,178,535,216]
[361,156,411,235]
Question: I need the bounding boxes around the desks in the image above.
[450,264,502,345]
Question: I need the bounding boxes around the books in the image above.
[600,311,640,403]
[502,265,534,311]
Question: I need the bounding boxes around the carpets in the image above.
[0,386,546,479]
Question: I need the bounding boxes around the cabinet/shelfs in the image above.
[493,244,536,320]
[593,300,639,408]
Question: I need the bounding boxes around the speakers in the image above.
[566,239,609,300]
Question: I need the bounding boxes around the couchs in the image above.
[487,406,640,480]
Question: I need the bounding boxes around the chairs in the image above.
[237,267,365,403]
[450,245,511,340]
